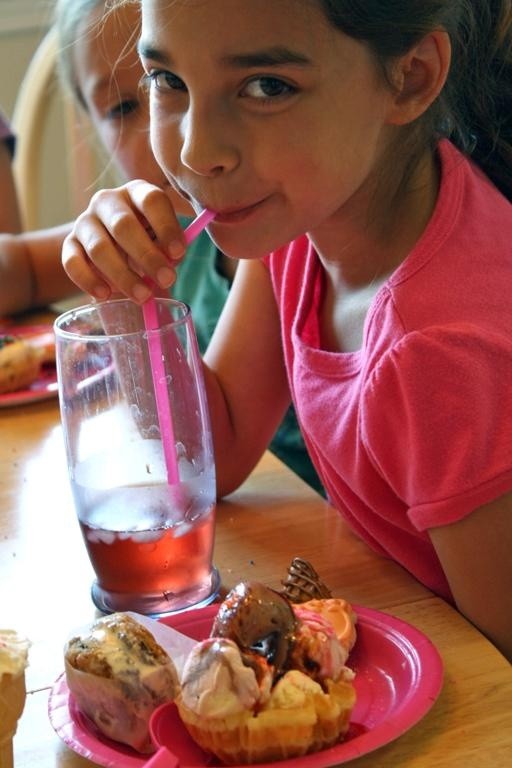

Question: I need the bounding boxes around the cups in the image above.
[54,296,222,619]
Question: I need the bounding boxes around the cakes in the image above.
[65,609,181,755]
[169,558,358,767]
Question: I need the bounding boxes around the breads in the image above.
[0,337,41,396]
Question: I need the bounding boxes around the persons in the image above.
[60,1,512,662]
[0,113,26,240]
[0,1,185,316]
[9,18,129,236]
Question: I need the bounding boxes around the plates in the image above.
[47,602,447,767]
[0,321,114,407]
